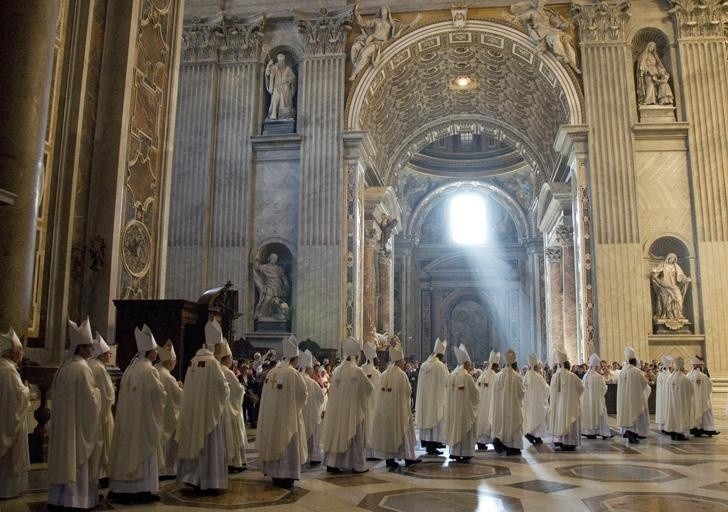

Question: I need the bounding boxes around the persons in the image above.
[650,252,691,319]
[652,69,675,105]
[348,3,409,81]
[0,315,720,512]
[253,253,290,323]
[510,0,582,75]
[636,41,667,106]
[265,53,297,119]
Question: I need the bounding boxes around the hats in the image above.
[296,346,313,369]
[89,329,110,358]
[214,331,234,361]
[155,337,177,363]
[434,337,448,356]
[387,344,405,362]
[363,340,378,360]
[340,334,362,357]
[453,343,470,363]
[132,322,158,351]
[204,317,223,345]
[66,313,93,348]
[282,333,299,359]
[0,326,23,352]
[488,345,705,366]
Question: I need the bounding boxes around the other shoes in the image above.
[449,454,474,464]
[587,434,596,438]
[602,435,614,440]
[623,430,647,444]
[689,428,718,438]
[421,440,446,455]
[552,442,576,452]
[385,461,400,468]
[404,458,421,467]
[525,433,536,446]
[491,438,521,456]
[477,442,487,450]
[661,430,689,441]
[535,437,543,445]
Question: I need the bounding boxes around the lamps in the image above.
[455,75,471,87]
